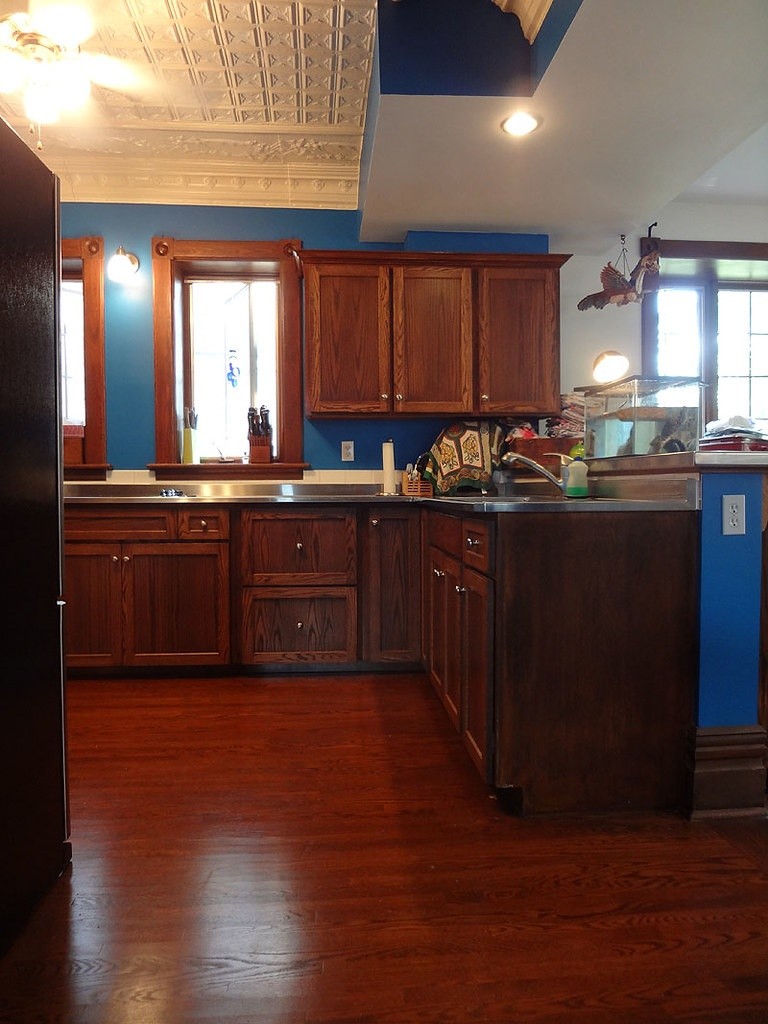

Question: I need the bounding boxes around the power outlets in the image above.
[721,494,747,536]
[340,440,355,463]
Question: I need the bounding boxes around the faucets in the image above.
[501,451,574,492]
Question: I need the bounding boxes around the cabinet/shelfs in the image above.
[236,505,364,585]
[298,247,477,420]
[475,251,577,417]
[233,580,363,673]
[362,507,419,672]
[423,512,461,825]
[454,514,703,823]
[57,505,235,673]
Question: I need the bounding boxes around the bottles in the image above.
[564,455,589,496]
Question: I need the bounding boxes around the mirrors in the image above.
[60,235,114,483]
[148,233,311,479]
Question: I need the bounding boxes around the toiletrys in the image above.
[564,453,591,499]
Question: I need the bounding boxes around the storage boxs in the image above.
[582,374,702,460]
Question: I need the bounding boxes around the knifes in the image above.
[248,405,271,434]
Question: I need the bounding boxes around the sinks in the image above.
[432,494,616,505]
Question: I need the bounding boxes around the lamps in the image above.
[105,243,140,286]
[0,14,69,72]
[591,350,630,384]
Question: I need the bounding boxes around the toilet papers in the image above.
[382,442,396,493]
